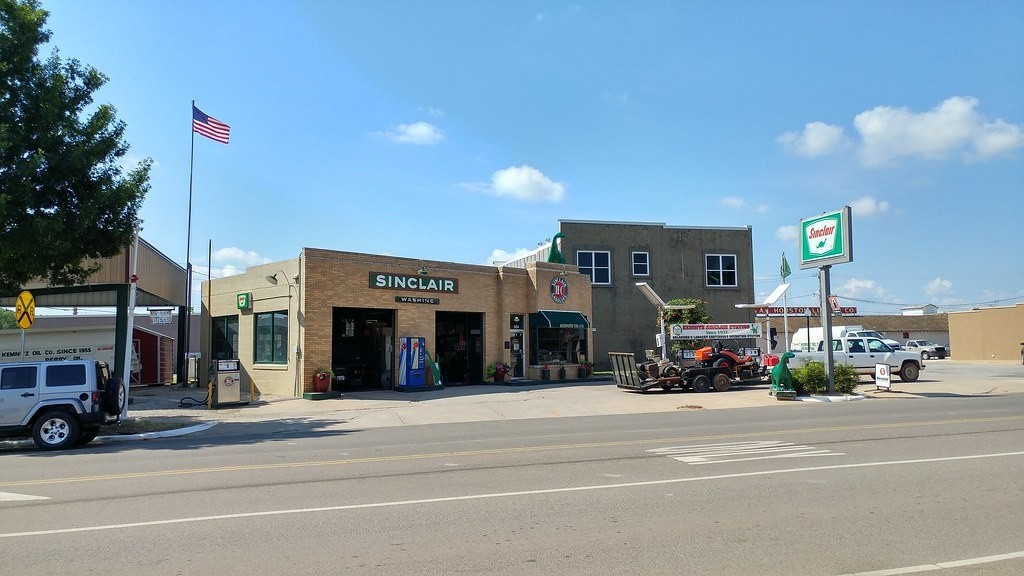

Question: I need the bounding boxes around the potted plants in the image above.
[542,355,550,381]
[578,360,592,378]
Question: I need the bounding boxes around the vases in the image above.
[494,371,504,383]
[312,374,330,392]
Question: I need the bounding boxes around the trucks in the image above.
[791,324,903,352]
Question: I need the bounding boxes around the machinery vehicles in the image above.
[608,340,779,393]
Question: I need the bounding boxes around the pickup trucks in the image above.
[901,339,947,360]
[786,336,926,382]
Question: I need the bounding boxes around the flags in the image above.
[193,105,231,145]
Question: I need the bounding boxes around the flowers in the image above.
[486,362,515,378]
[314,367,335,379]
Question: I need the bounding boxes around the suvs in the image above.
[1,360,127,451]
